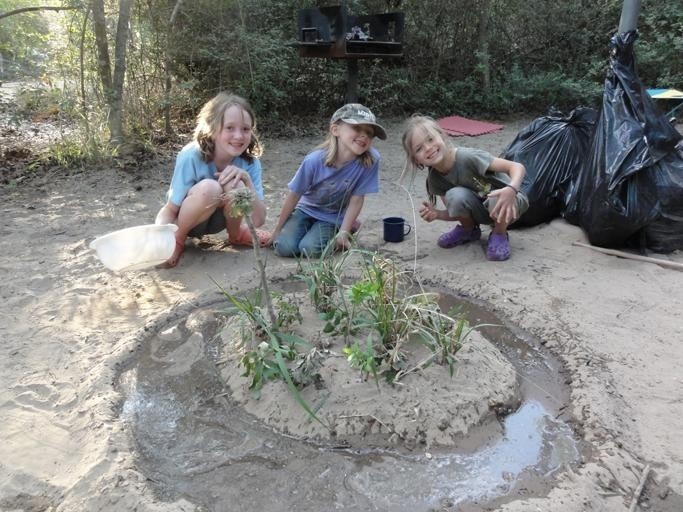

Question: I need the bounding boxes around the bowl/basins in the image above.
[90,223,179,273]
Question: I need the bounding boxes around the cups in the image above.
[383,216,411,242]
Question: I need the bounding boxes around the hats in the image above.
[329,103,387,140]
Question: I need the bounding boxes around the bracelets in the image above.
[508,184,518,195]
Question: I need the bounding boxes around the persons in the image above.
[153,92,273,271]
[265,103,388,260]
[401,114,530,262]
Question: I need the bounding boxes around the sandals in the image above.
[166,240,184,266]
[228,226,272,247]
[438,225,482,249]
[485,232,512,261]
